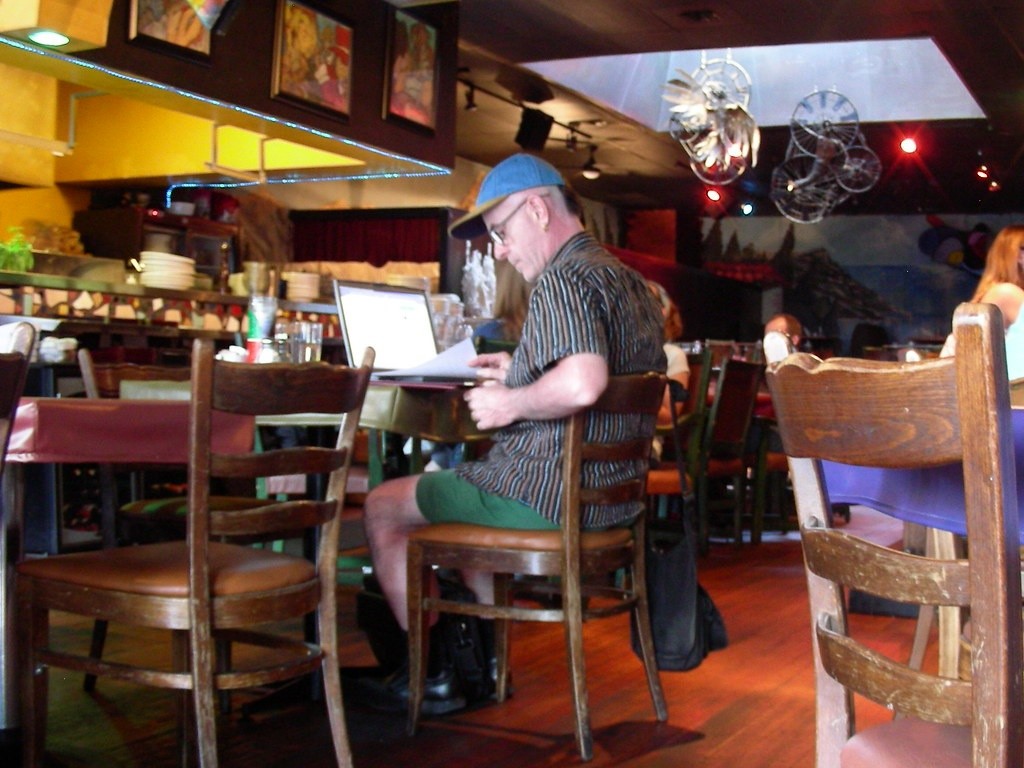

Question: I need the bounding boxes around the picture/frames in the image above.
[269,0,354,123]
[381,5,442,138]
[128,0,213,69]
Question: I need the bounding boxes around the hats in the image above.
[447,154,567,241]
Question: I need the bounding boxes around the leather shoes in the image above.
[345,659,465,712]
[479,660,514,701]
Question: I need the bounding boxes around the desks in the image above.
[121,379,500,733]
[817,405,1024,721]
[6,395,248,715]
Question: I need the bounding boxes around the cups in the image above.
[247,339,263,363]
[243,261,268,296]
[290,321,311,363]
[311,324,323,362]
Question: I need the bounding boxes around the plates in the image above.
[281,273,320,303]
[129,251,196,290]
[390,277,465,355]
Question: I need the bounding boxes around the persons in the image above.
[350,150,692,730]
[762,313,801,344]
[939,223,1024,381]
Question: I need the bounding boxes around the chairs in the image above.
[1,302,1024,768]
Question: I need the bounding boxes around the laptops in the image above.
[332,279,482,386]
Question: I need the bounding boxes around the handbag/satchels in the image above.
[630,511,727,671]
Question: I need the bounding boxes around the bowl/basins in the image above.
[166,202,195,216]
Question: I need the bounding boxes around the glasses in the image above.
[488,192,550,243]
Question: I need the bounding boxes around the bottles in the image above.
[263,340,274,363]
[278,340,287,363]
[461,243,497,319]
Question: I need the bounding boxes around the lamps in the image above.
[515,109,553,150]
[566,137,577,151]
[464,89,477,112]
[581,146,600,179]
